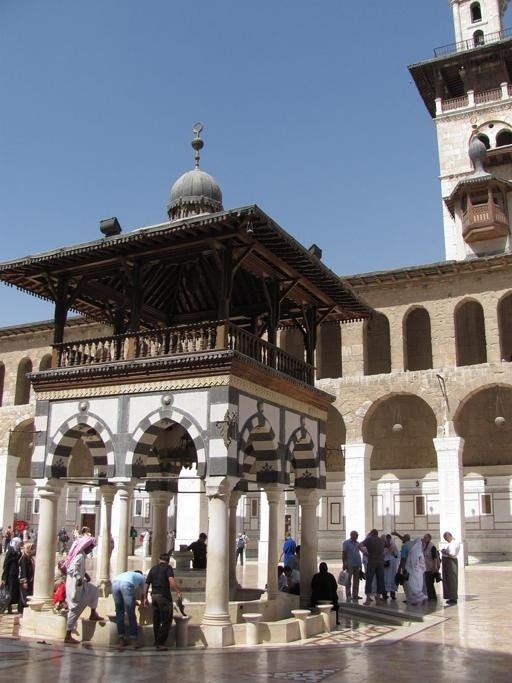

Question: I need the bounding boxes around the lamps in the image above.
[389,396,409,432]
[492,385,509,427]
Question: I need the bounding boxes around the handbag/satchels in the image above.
[337,571,350,586]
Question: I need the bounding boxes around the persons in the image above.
[112,569,145,648]
[54,524,252,569]
[53,578,70,615]
[275,529,461,606]
[61,534,104,643]
[310,561,340,624]
[54,560,68,592]
[0,520,39,614]
[143,552,188,650]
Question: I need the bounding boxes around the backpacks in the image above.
[238,538,245,549]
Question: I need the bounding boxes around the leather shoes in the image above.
[119,639,128,647]
[132,640,146,649]
